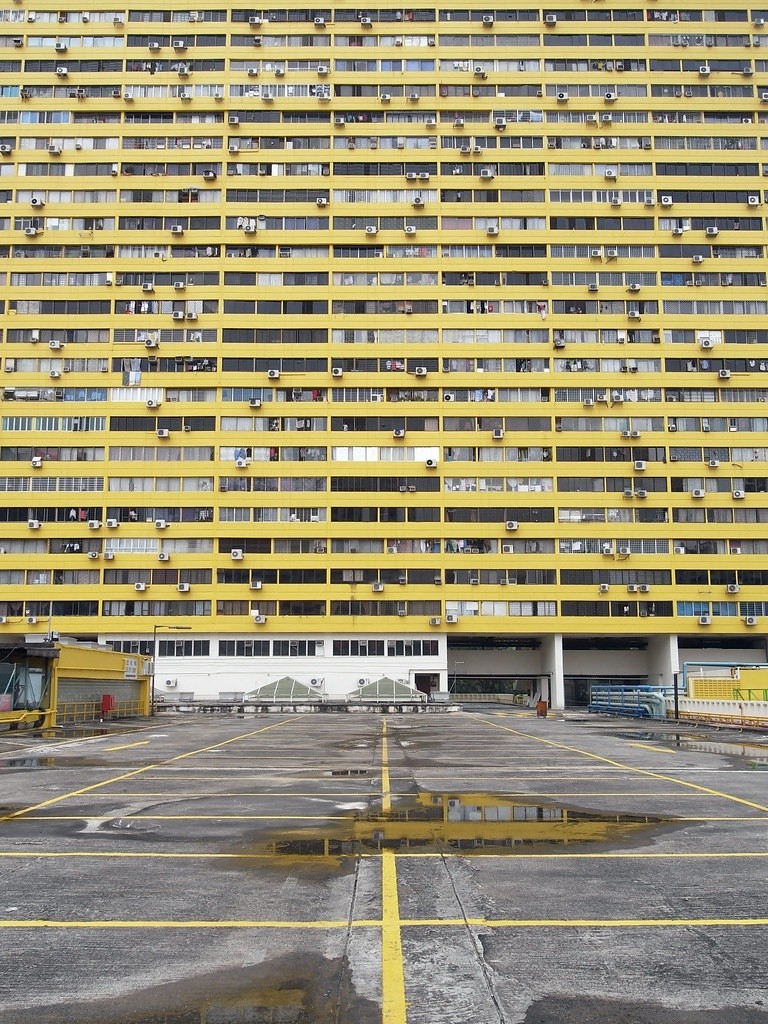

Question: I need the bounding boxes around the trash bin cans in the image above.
[537,701,547,719]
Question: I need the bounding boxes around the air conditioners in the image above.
[165,678,177,687]
[309,677,322,686]
[144,662,155,675]
[373,831,383,840]
[0,16,768,647]
[357,677,369,686]
[431,796,442,804]
[448,799,459,808]
[474,839,483,845]
[511,838,523,847]
